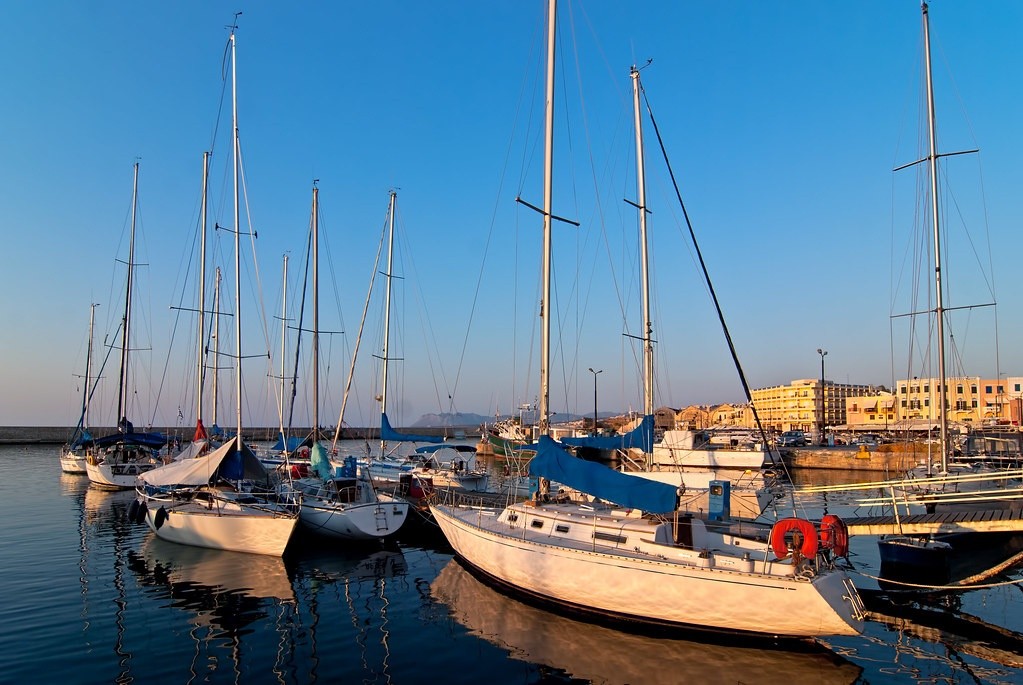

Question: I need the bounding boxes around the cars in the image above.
[819,438,843,446]
[855,434,884,446]
[776,430,807,447]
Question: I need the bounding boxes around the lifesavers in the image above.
[770,517,819,559]
[88,455,95,465]
[820,514,848,556]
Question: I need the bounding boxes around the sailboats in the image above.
[869,0,1022,514]
[61,470,864,685]
[59,0,871,640]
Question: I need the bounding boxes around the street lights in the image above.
[817,348,828,447]
[588,367,602,438]
